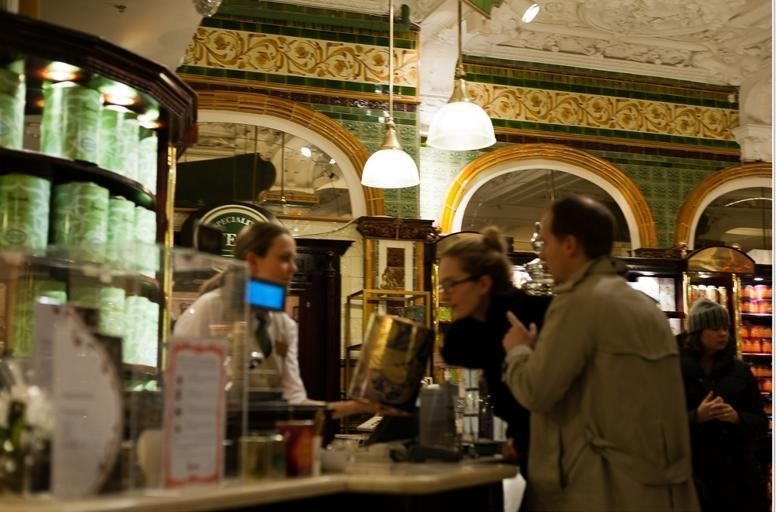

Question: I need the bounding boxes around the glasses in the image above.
[436,272,485,293]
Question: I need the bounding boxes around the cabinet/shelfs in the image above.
[736,264,772,436]
[0,11,201,393]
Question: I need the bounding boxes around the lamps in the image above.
[425,0,497,152]
[360,0,421,189]
[503,0,541,24]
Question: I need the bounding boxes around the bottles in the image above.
[462,386,493,438]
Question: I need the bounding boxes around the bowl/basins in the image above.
[318,444,354,474]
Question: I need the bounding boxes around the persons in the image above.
[501,192,702,510]
[434,220,554,511]
[166,220,413,423]
[674,297,772,512]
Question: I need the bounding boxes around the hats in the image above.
[686,297,731,334]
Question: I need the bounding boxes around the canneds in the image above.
[0,271,158,393]
[55,180,159,282]
[238,431,287,480]
[687,283,772,433]
[0,69,26,151]
[41,79,156,195]
[345,311,436,410]
[0,172,51,253]
[274,419,314,478]
